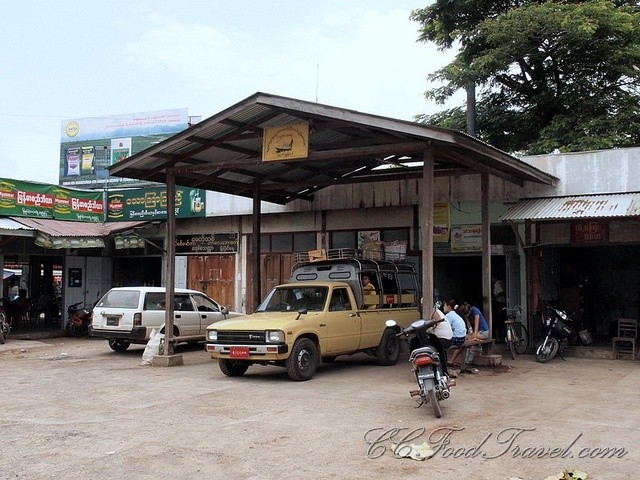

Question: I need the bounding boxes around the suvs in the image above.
[87,287,247,353]
[204,259,420,380]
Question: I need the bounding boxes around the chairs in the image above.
[613,318,637,360]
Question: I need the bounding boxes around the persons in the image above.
[491,273,506,344]
[361,272,377,310]
[446,301,490,364]
[442,296,467,346]
[417,297,453,349]
[9,288,29,328]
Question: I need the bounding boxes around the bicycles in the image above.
[502,307,529,359]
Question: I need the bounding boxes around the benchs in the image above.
[445,337,494,371]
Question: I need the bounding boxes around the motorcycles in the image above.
[536,309,579,362]
[65,301,92,339]
[0,312,12,344]
[396,318,456,417]
